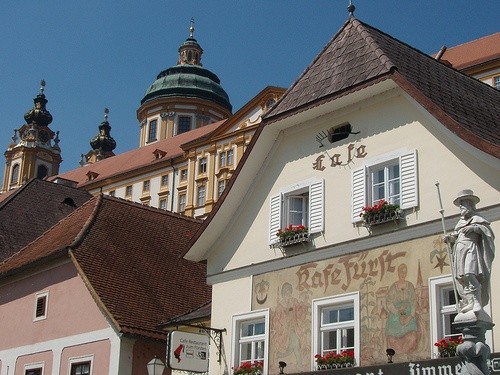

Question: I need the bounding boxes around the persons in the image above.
[442,189,495,314]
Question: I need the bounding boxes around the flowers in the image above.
[276,223,308,240]
[232,362,263,375]
[315,350,354,369]
[434,336,464,358]
[359,200,400,218]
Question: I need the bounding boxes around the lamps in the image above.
[386,349,395,363]
[279,361,287,374]
